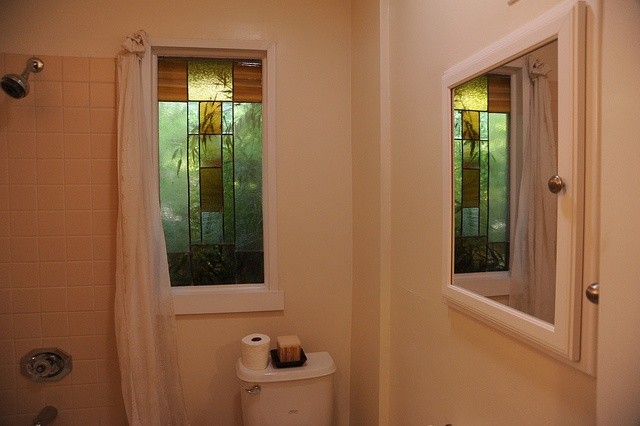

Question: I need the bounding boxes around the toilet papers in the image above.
[241,334,271,370]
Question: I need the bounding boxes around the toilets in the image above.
[237,351,337,425]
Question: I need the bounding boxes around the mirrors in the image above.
[138,33,287,320]
[436,0,586,365]
